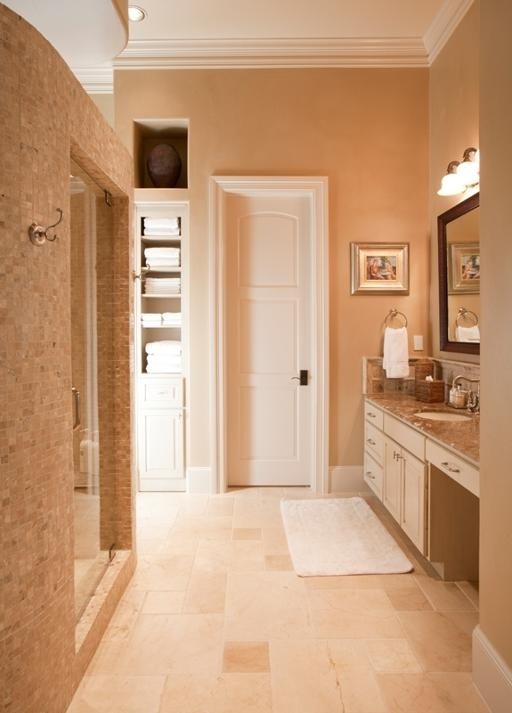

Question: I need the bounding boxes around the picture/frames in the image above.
[437,191,480,354]
[447,240,480,295]
[350,242,410,296]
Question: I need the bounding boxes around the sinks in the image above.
[411,408,471,423]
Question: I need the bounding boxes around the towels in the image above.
[141,218,182,372]
[382,327,409,379]
[455,326,481,344]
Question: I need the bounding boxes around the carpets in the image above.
[280,496,414,577]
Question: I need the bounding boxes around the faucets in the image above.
[452,375,480,390]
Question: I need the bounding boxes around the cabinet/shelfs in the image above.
[363,401,479,581]
[134,200,189,494]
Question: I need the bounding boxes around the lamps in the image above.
[436,147,481,196]
